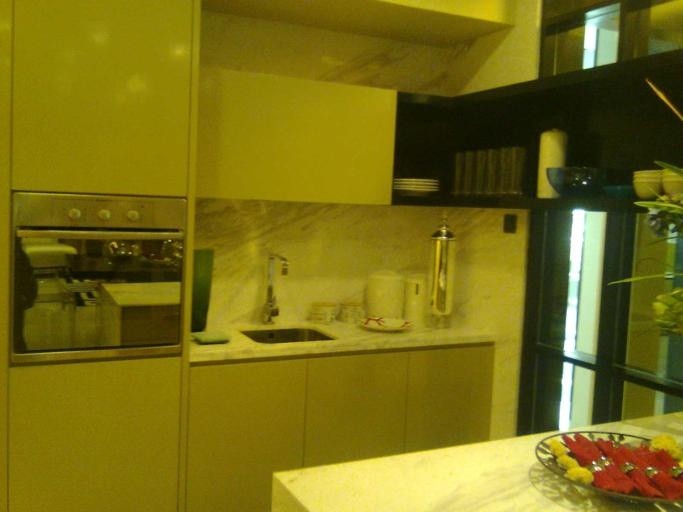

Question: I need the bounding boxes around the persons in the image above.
[15,234,38,353]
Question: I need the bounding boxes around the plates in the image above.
[356,316,414,332]
[535,431,680,506]
[393,179,439,197]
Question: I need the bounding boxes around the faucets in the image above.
[261,253,290,322]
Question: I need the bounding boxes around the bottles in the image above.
[535,126,568,199]
[368,268,405,318]
[404,273,429,333]
[425,213,458,331]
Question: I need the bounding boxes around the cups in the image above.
[339,303,366,324]
[311,302,337,324]
[451,147,525,195]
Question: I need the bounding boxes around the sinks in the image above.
[240,326,337,345]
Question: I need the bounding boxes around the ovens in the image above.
[9,191,189,366]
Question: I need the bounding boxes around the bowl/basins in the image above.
[632,169,683,200]
[546,167,598,197]
[602,185,634,199]
